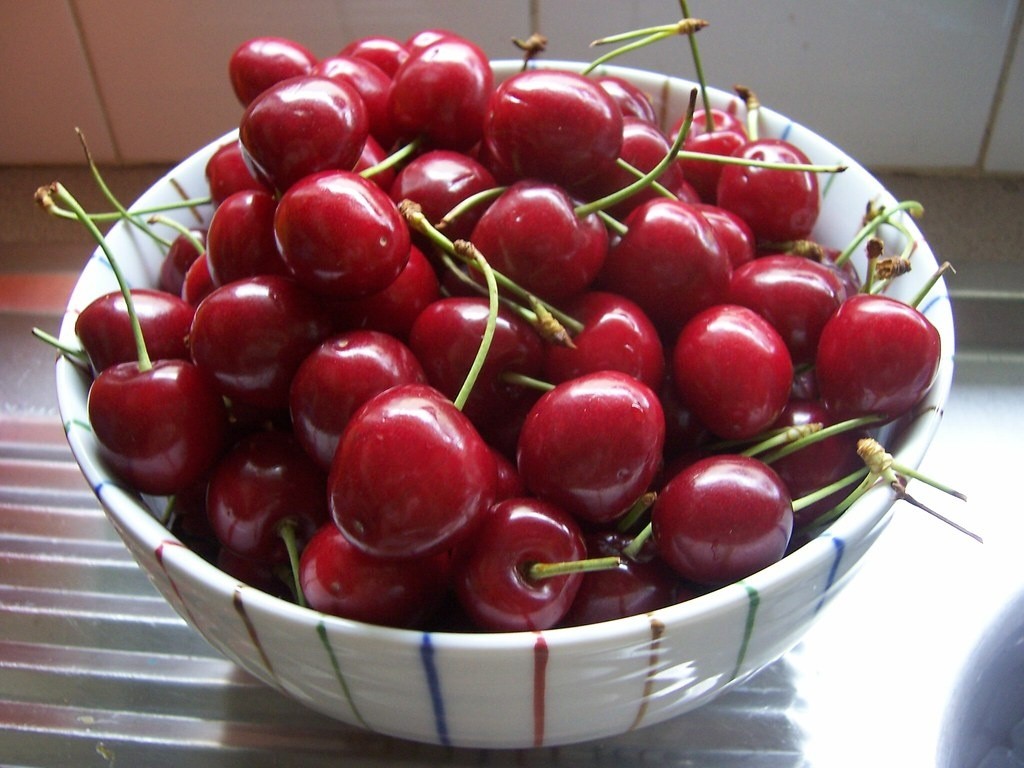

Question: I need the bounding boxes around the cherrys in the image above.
[74,31,941,633]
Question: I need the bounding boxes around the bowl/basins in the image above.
[54,57,956,750]
